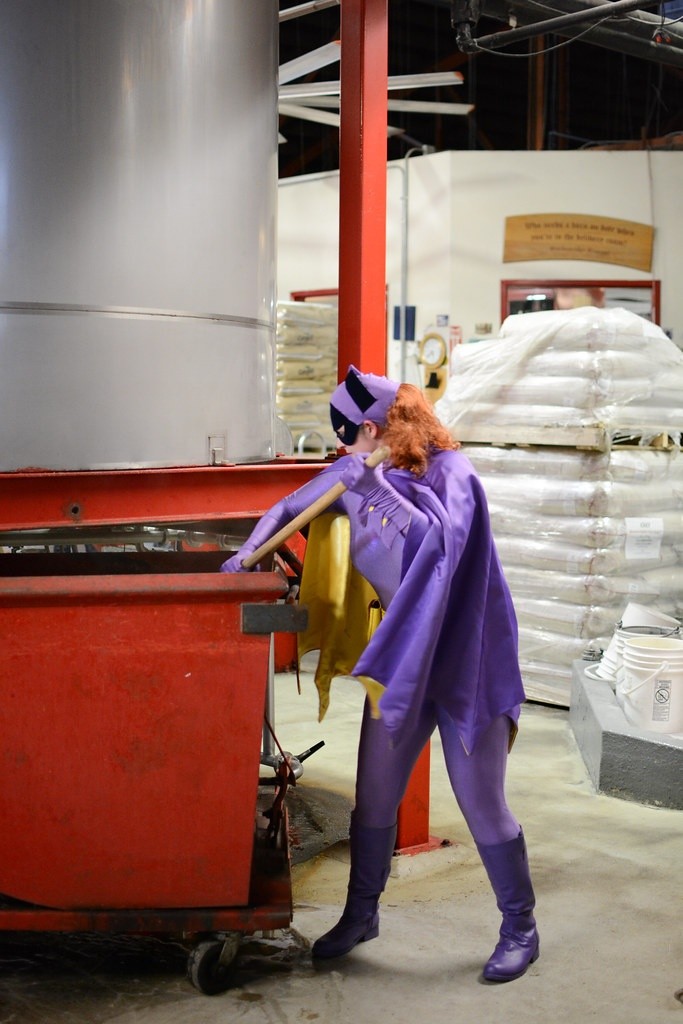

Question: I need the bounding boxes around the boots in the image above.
[311,810,397,958]
[474,824,539,982]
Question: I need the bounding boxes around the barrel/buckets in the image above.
[615,627,683,703]
[622,638,683,734]
[584,601,682,681]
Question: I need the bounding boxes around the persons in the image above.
[217,366,541,983]
